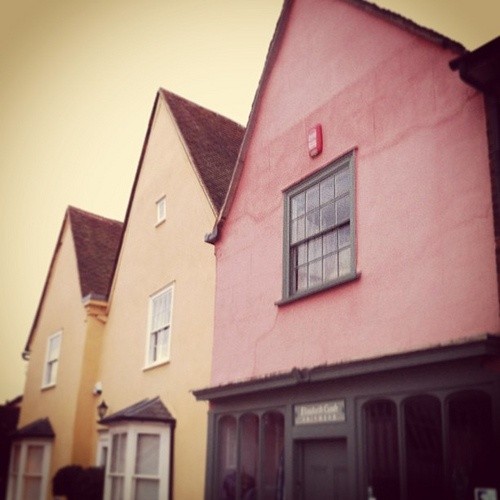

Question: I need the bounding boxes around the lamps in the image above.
[95,400,108,425]
